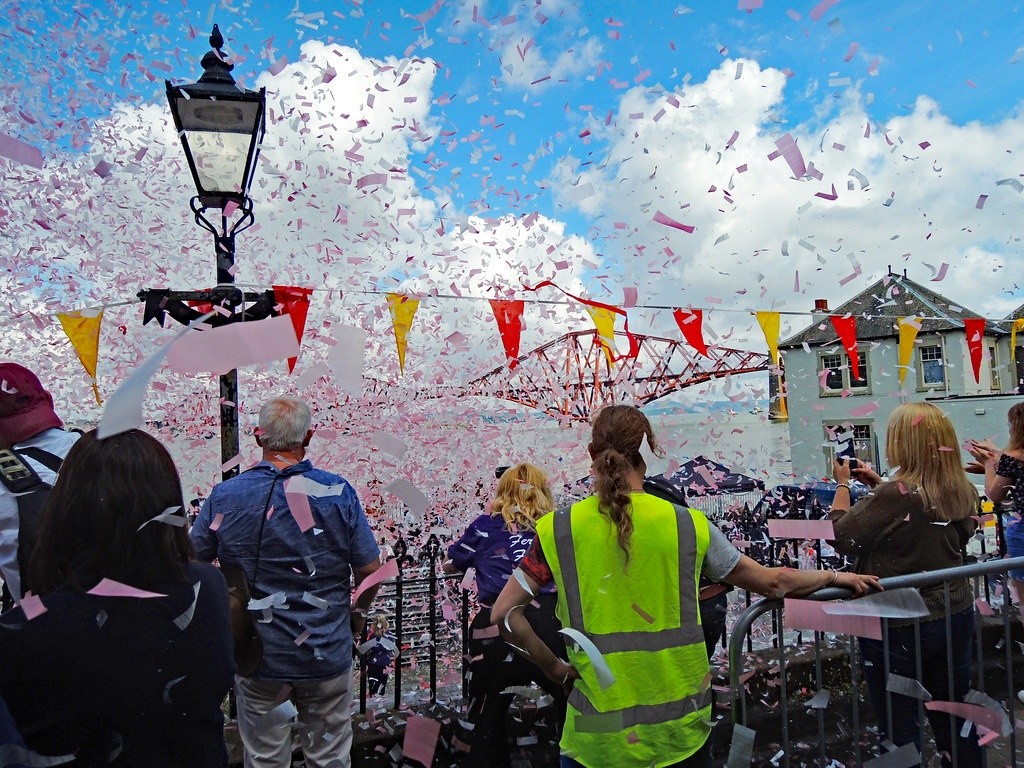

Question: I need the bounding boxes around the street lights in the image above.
[163,24,270,481]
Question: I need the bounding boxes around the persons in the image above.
[0,426,234,768]
[964,401,1024,616]
[443,464,573,768]
[366,615,392,702]
[491,404,886,768]
[191,397,381,768]
[1,362,96,606]
[823,401,987,768]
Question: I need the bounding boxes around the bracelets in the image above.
[835,484,851,494]
[829,568,838,586]
[827,569,839,586]
[835,484,852,494]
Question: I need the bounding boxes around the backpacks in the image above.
[0,427,89,593]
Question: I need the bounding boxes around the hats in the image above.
[0,362,63,444]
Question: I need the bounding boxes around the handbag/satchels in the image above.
[221,566,253,660]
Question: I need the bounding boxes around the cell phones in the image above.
[838,438,859,477]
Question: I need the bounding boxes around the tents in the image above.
[647,454,766,496]
[562,471,599,500]
